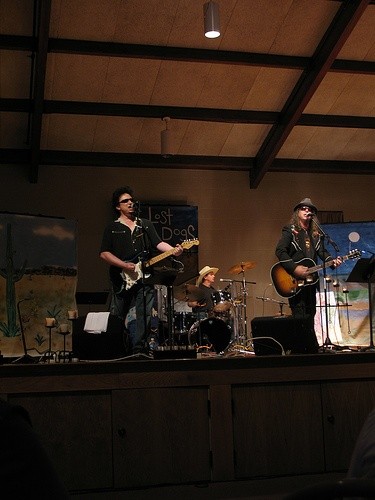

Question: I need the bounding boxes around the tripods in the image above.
[11,298,40,364]
[223,280,256,357]
[311,216,375,352]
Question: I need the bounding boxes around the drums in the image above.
[171,289,237,354]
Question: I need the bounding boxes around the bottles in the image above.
[149,338,157,358]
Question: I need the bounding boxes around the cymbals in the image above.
[228,259,257,274]
[172,284,210,302]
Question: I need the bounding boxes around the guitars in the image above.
[109,237,200,301]
[270,248,361,299]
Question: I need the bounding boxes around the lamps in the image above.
[203,1,221,38]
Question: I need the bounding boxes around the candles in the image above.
[68,311,77,319]
[46,317,55,326]
[61,324,68,332]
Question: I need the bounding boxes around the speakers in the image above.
[251,315,320,355]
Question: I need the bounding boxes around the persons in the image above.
[347,406,375,483]
[187,265,235,351]
[274,198,343,353]
[0,400,69,500]
[125,302,159,345]
[97,186,183,358]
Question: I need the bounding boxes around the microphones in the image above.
[306,213,315,217]
[219,278,233,282]
[131,201,138,208]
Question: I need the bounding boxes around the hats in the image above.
[197,266,219,286]
[294,198,317,214]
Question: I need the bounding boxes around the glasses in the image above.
[119,198,132,203]
[299,206,312,212]
